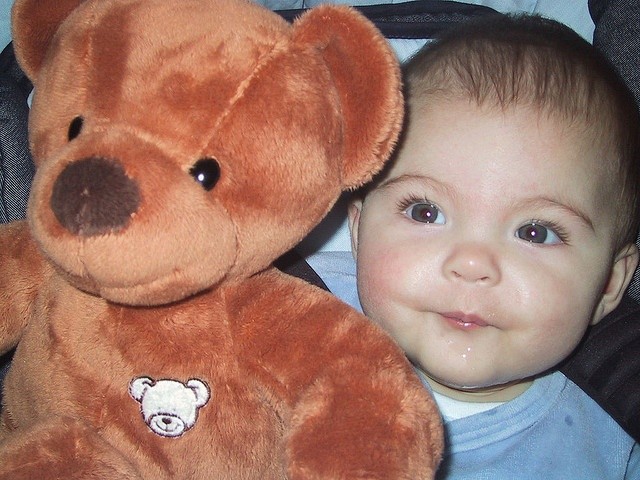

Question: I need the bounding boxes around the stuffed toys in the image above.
[1,0,445,480]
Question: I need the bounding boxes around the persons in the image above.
[294,14,639,479]
